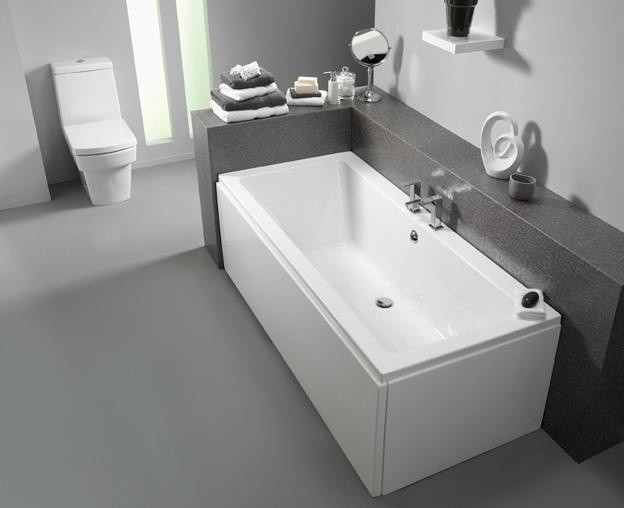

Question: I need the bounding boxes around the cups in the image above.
[509,173,537,202]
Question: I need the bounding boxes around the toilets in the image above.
[49,55,139,205]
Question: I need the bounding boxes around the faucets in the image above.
[405,194,444,231]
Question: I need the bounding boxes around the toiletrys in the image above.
[336,65,357,102]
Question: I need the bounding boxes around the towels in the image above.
[208,59,330,124]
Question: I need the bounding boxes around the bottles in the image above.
[337,67,356,99]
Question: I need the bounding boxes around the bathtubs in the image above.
[215,151,563,500]
